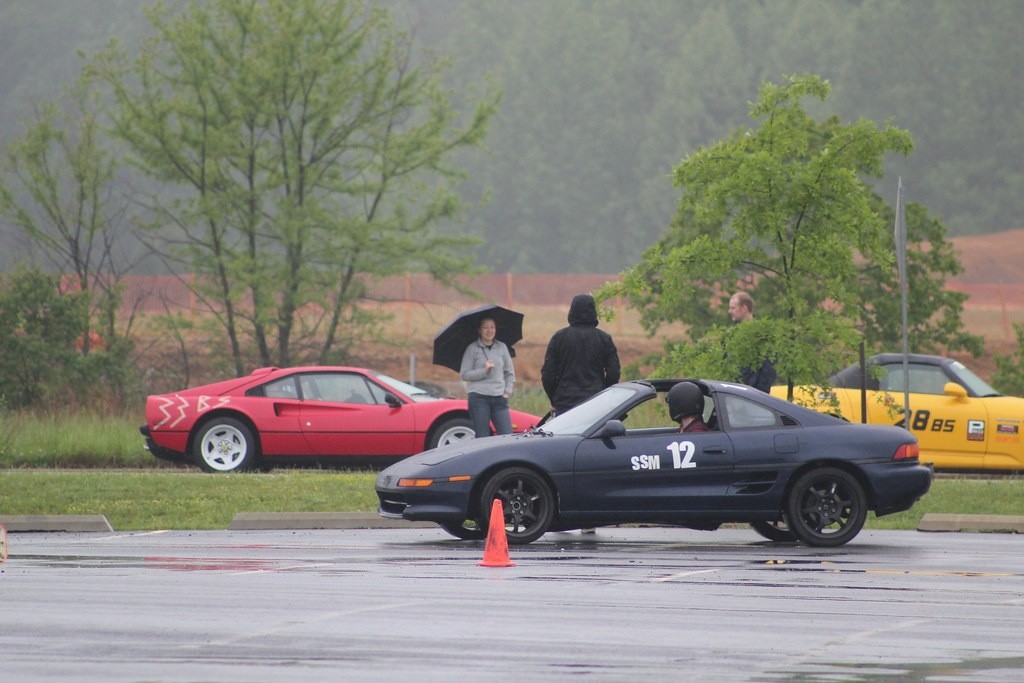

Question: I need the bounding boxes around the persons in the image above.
[460,315,515,437]
[664,381,711,433]
[721,292,776,395]
[540,295,621,533]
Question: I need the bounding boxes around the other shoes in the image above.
[581,527,595,533]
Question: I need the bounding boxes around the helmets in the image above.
[665,381,704,421]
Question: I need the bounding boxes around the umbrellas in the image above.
[433,304,524,373]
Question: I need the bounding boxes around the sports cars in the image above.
[138,365,546,474]
[374,377,937,546]
[769,351,1024,476]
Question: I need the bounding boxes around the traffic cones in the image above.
[477,497,517,567]
